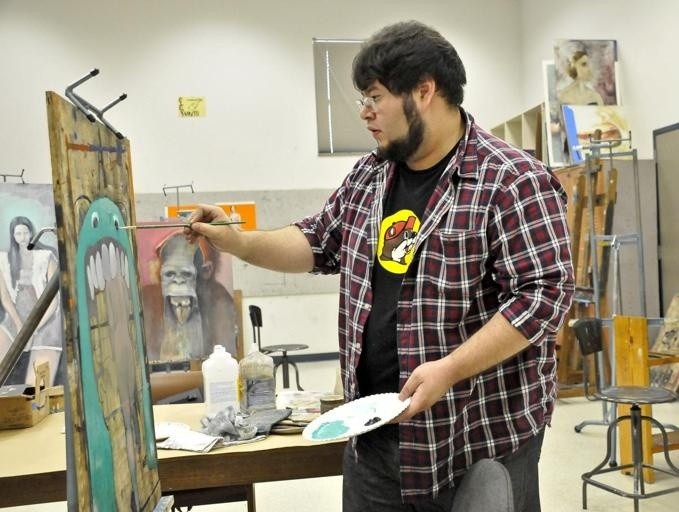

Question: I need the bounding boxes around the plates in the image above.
[302,391,412,444]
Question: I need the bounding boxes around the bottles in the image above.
[200,344,239,412]
[235,344,277,415]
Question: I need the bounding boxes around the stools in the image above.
[581,384,678,512]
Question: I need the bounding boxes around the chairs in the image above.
[248,304,310,392]
[564,317,678,470]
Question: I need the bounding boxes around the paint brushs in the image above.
[120,221,246,230]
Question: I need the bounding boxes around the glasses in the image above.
[355,90,392,114]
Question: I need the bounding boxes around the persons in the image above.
[553,51,604,163]
[180,18,577,512]
[0,216,63,386]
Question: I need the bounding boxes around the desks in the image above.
[0,399,361,512]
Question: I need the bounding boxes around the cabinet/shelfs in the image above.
[487,103,552,166]
